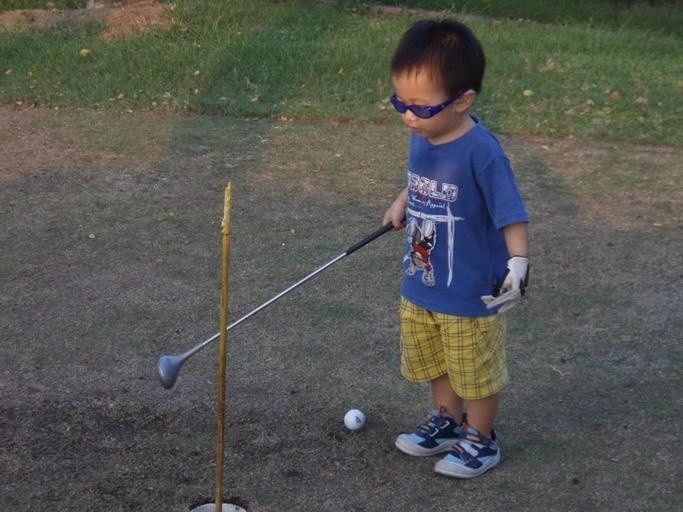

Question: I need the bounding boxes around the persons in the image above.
[382,19,530,480]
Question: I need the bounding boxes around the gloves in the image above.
[481,255,531,317]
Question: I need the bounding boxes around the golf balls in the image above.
[343,408,366,431]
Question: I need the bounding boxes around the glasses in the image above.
[386,91,466,119]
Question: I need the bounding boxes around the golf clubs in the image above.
[158,214,407,389]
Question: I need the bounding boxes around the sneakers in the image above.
[432,422,502,479]
[392,401,467,459]
